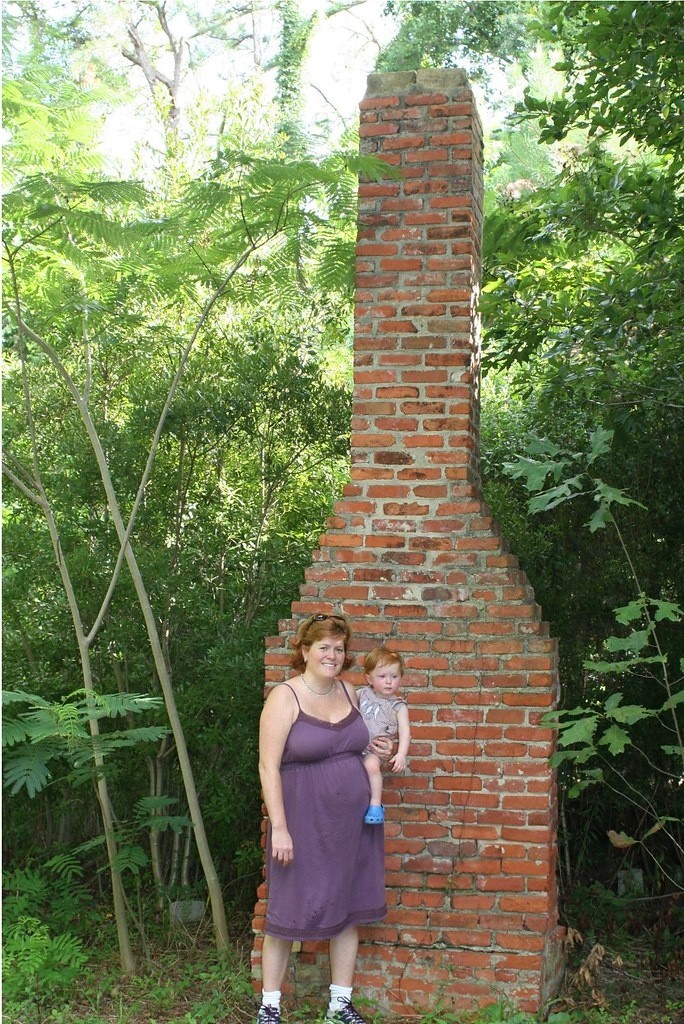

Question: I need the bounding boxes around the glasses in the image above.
[306,613,346,632]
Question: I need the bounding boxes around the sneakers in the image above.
[323,995,366,1024]
[257,1004,282,1024]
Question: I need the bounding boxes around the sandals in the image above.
[363,802,385,825]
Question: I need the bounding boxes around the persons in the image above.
[259,613,394,1024]
[357,647,410,824]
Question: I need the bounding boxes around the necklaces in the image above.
[301,674,336,696]
